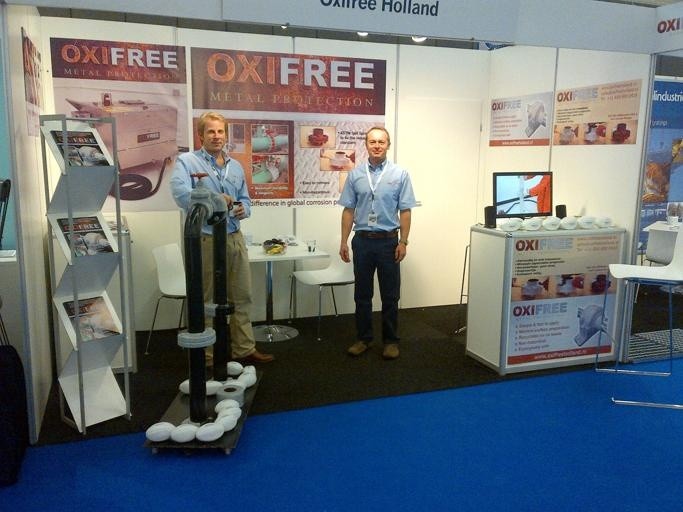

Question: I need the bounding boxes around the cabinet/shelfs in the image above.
[38,115,136,435]
[466,224,627,375]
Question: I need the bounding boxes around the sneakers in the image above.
[348,341,373,356]
[383,343,399,357]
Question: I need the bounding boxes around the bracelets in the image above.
[398,239,408,245]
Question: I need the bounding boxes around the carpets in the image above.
[36,303,615,443]
[628,328,683,364]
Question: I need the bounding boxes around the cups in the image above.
[666,215,679,226]
[306,239,316,252]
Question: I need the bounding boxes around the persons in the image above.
[169,111,276,369]
[338,125,418,360]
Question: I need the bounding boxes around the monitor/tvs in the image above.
[492,171,553,218]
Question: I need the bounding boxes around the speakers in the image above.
[484,206,496,228]
[556,205,566,219]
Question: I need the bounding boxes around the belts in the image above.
[355,229,399,239]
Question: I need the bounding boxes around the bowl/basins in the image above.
[265,244,287,256]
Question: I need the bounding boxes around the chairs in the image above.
[596,202,683,409]
[143,235,355,354]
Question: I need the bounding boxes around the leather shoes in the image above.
[245,349,273,362]
